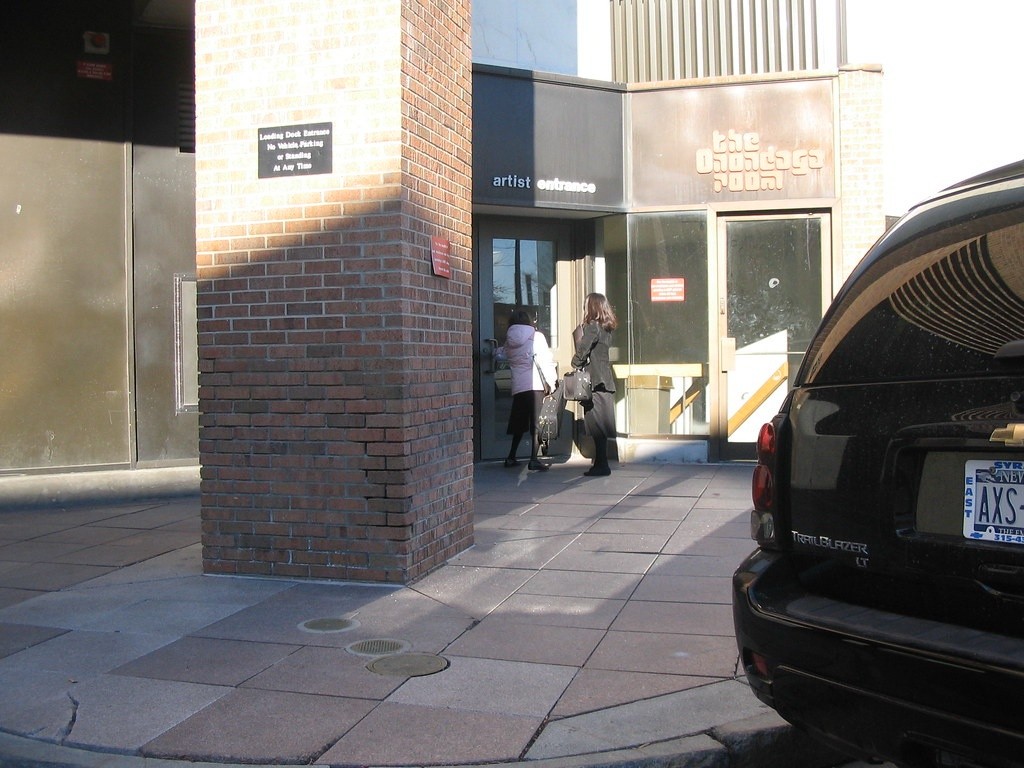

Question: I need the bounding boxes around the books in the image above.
[572,324,590,367]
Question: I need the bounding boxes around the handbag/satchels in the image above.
[564,369,593,402]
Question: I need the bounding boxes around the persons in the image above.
[491,311,557,470]
[571,293,617,477]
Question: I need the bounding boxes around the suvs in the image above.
[731,157,1023,768]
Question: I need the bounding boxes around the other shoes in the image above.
[504,460,521,467]
[528,463,550,471]
[585,467,612,476]
